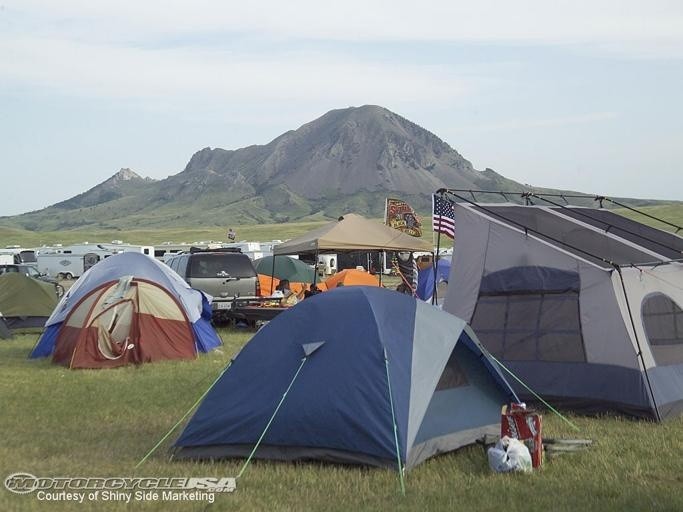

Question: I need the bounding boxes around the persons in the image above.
[300,283,322,300]
[279,278,308,301]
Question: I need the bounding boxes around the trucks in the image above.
[0,239,453,300]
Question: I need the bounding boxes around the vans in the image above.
[162,247,261,327]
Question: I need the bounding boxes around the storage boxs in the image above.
[501,405,542,468]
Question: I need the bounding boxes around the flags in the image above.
[432,191,455,238]
[386,196,423,237]
[227,227,236,242]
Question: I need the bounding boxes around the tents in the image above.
[26,248,222,373]
[433,184,683,423]
[250,255,326,297]
[415,255,450,304]
[326,267,385,289]
[133,283,579,496]
[0,270,59,337]
